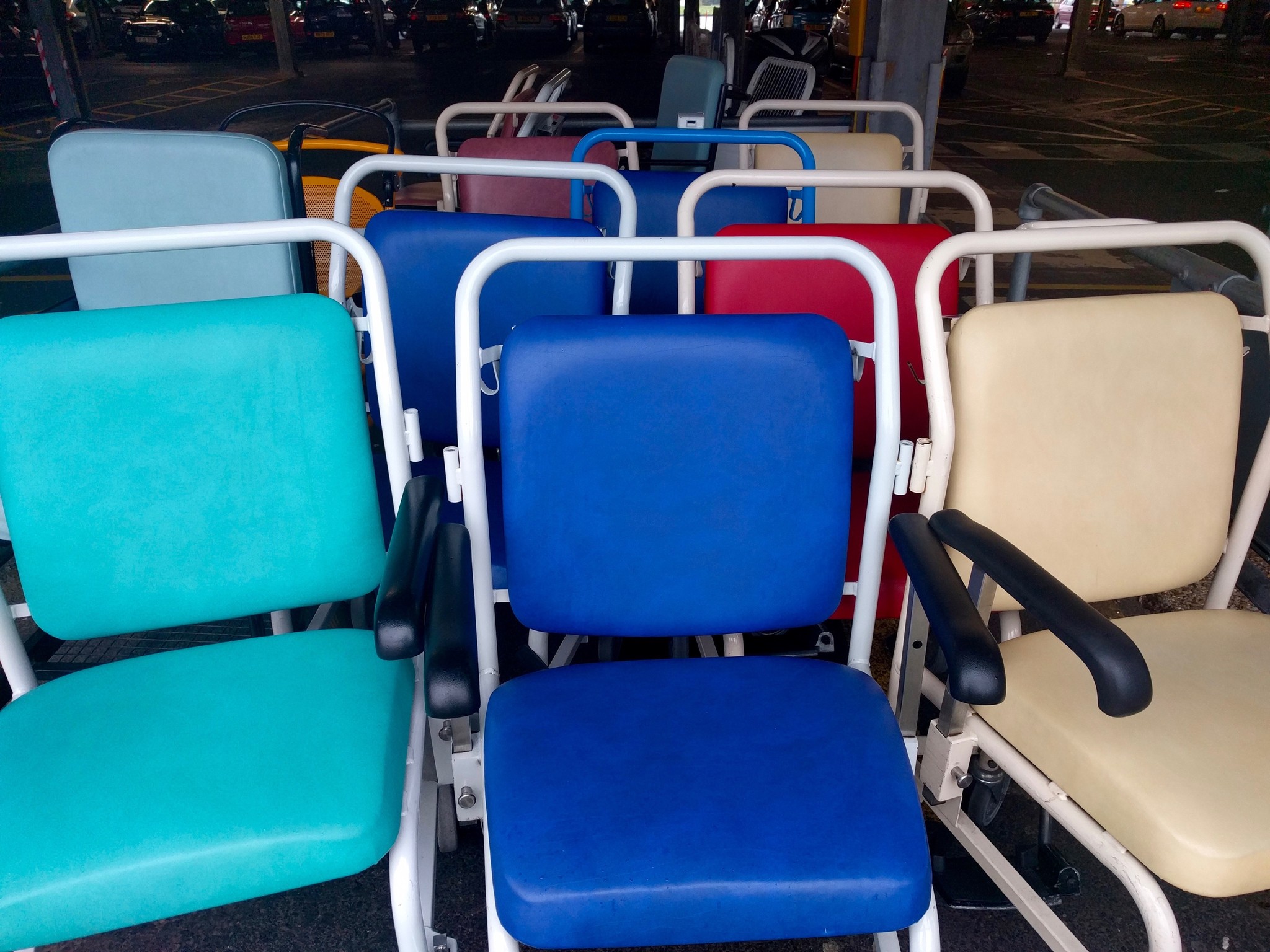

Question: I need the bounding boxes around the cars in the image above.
[582,0,660,52]
[495,0,578,52]
[567,0,585,19]
[1053,0,1121,30]
[1215,0,1270,42]
[222,0,307,55]
[751,0,837,40]
[826,0,974,66]
[407,0,499,50]
[0,0,228,119]
[958,0,1056,43]
[1111,0,1227,40]
[302,0,411,56]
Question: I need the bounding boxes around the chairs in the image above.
[0,56,1270,952]
